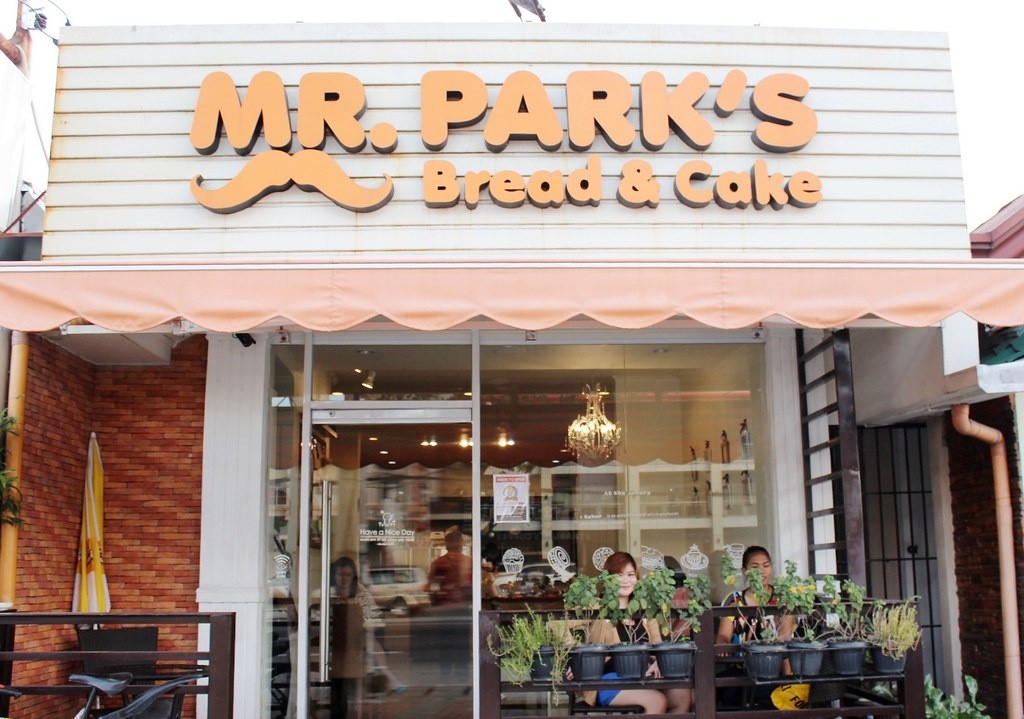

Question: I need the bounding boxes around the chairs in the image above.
[77,626,174,719]
[564,628,645,715]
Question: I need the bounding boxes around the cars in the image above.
[307,565,434,627]
[492,562,577,599]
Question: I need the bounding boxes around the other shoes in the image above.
[392,683,407,693]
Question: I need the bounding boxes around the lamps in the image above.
[359,370,377,389]
[568,378,622,458]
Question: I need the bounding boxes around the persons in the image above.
[311,555,409,697]
[715,544,794,677]
[569,549,697,715]
[427,529,477,700]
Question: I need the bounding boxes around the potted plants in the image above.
[486,601,582,706]
[604,568,676,679]
[562,569,624,680]
[774,575,826,675]
[866,595,924,674]
[650,573,712,678]
[722,553,798,679]
[819,575,870,674]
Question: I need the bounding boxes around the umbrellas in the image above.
[70,429,111,633]
